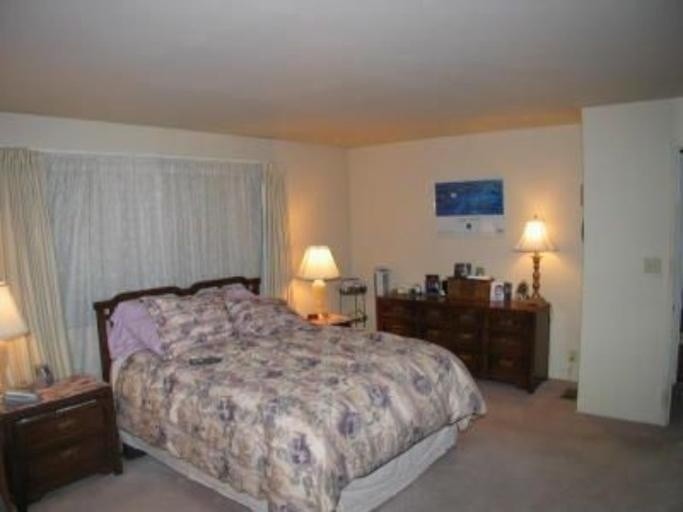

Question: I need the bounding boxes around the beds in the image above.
[94,274,484,512]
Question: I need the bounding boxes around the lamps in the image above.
[0,283,27,342]
[294,247,339,314]
[511,219,555,306]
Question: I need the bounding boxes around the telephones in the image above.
[3,391,40,406]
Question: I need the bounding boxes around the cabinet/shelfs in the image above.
[0,374,126,512]
[377,292,549,394]
